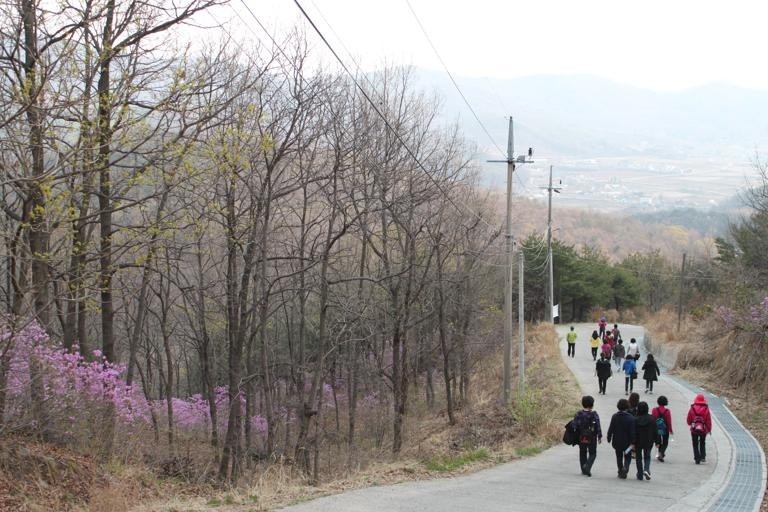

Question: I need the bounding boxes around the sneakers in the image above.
[618,468,651,480]
[657,453,666,460]
[695,458,705,464]
[581,464,592,476]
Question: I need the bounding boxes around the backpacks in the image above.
[656,407,669,435]
[691,405,706,433]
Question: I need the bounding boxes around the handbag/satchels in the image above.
[590,422,597,434]
[631,368,637,379]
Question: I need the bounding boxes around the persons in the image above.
[573,395,603,477]
[687,393,712,464]
[593,352,611,395]
[608,393,673,481]
[589,317,640,373]
[565,326,577,359]
[622,355,637,395]
[641,353,661,395]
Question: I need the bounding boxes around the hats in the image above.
[694,395,707,404]
